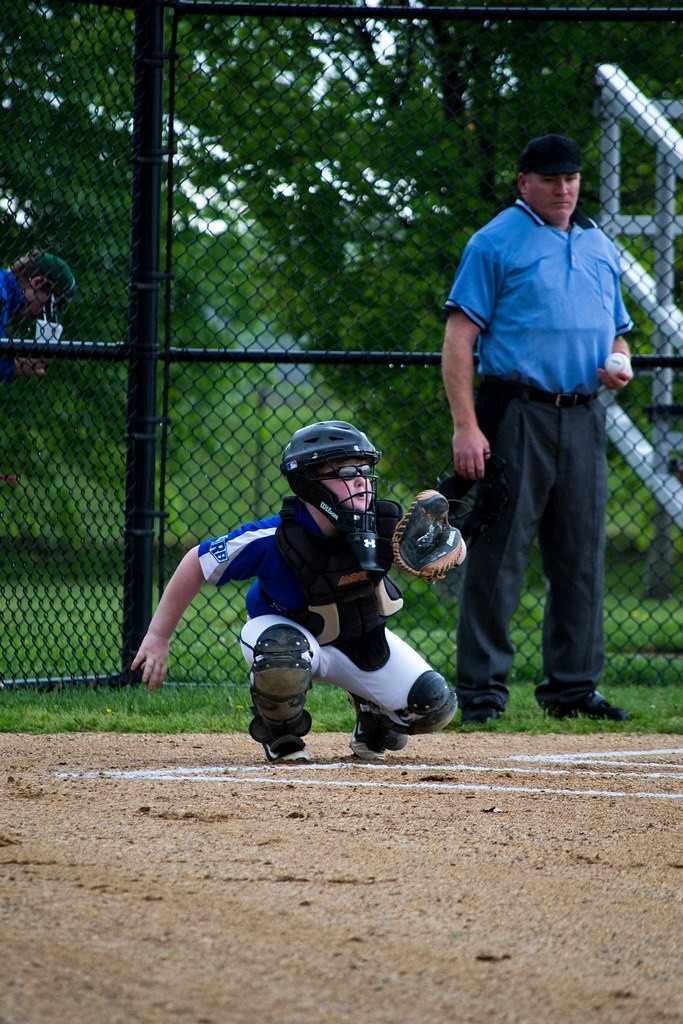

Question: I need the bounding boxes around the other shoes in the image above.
[461,706,500,724]
[548,698,625,721]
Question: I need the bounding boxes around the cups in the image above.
[35,319,63,342]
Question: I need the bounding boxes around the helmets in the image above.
[280,420,385,571]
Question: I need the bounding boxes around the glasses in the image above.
[318,464,371,480]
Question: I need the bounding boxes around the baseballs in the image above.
[604,352,632,378]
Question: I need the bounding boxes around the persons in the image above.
[439,135,633,731]
[0,247,78,384]
[131,420,467,763]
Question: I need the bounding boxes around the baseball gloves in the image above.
[390,489,467,586]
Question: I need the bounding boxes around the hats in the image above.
[518,135,581,176]
[30,253,77,303]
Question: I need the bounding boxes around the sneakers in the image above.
[348,691,385,761]
[262,741,309,764]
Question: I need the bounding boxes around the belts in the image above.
[481,375,597,409]
